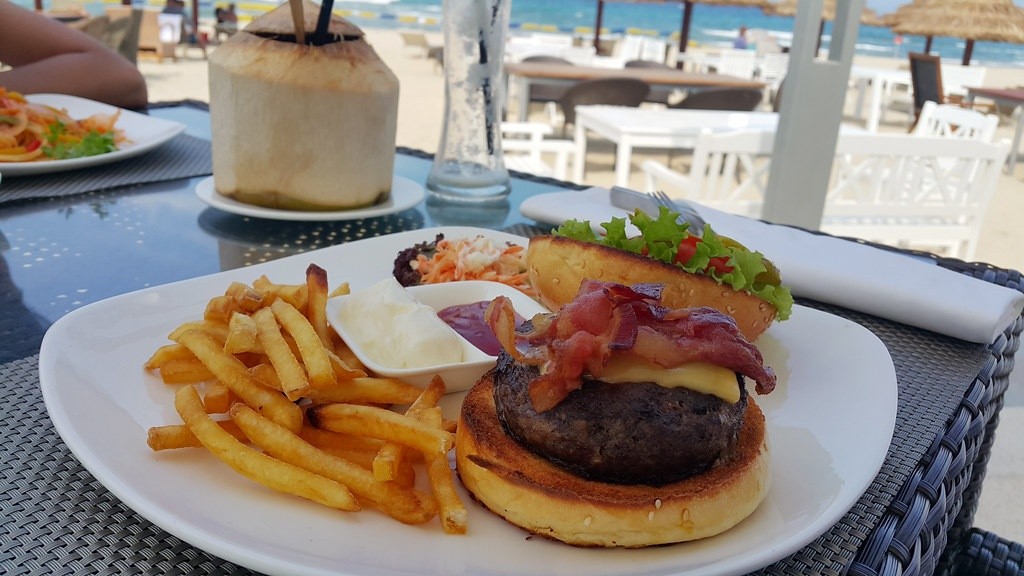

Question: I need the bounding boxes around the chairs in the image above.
[488,105,579,187]
[504,19,1014,97]
[876,99,1000,211]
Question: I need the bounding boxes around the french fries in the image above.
[144,264,471,535]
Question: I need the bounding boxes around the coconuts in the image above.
[208,0,401,210]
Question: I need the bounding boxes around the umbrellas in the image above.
[649,0,1024,73]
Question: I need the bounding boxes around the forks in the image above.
[645,188,697,236]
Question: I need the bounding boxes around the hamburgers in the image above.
[454,194,797,546]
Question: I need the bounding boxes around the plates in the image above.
[37,222,901,574]
[0,93,186,175]
[194,174,424,223]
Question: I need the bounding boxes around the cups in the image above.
[426,1,512,205]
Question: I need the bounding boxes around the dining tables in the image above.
[1,97,1024,575]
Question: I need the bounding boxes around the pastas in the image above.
[0,82,131,163]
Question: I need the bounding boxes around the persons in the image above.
[162,0,238,60]
[0,0,148,108]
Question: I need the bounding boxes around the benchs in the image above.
[640,129,1012,263]
